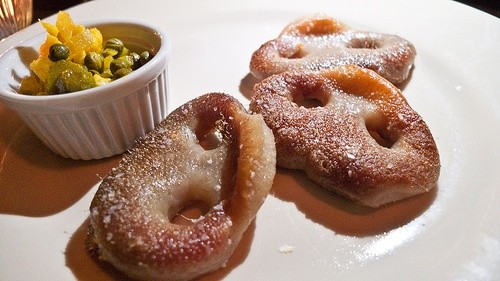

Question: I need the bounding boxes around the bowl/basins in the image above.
[0,16,172,159]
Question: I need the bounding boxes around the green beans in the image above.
[48,38,154,81]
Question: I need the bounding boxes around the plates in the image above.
[0,0,499,281]
